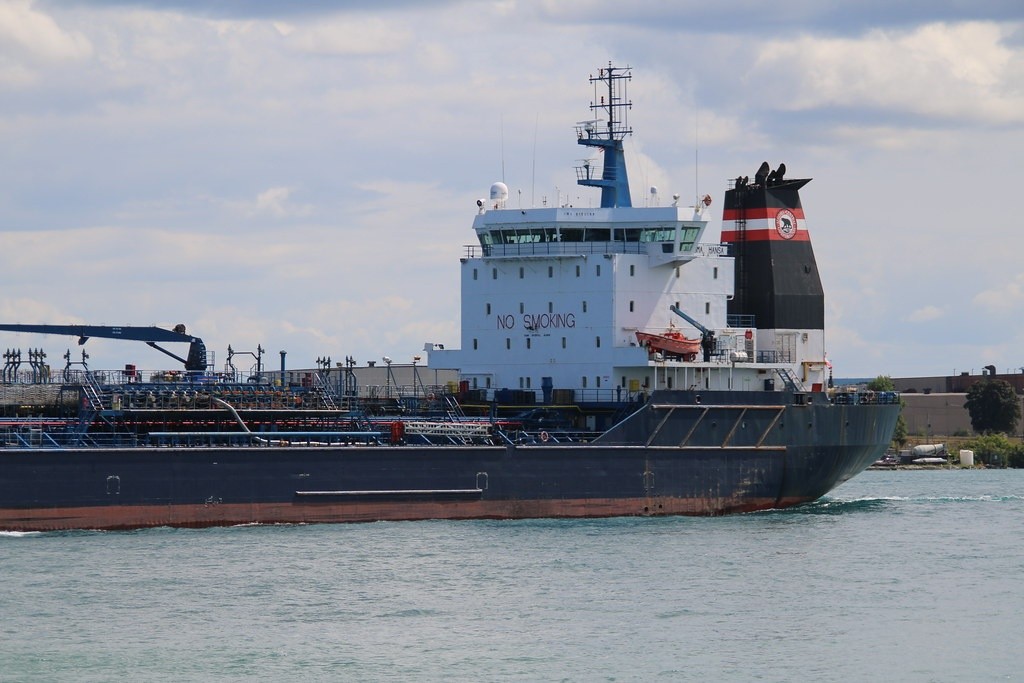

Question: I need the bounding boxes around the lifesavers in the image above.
[426,392,435,402]
[541,430,549,441]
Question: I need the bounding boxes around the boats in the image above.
[2,386,901,529]
